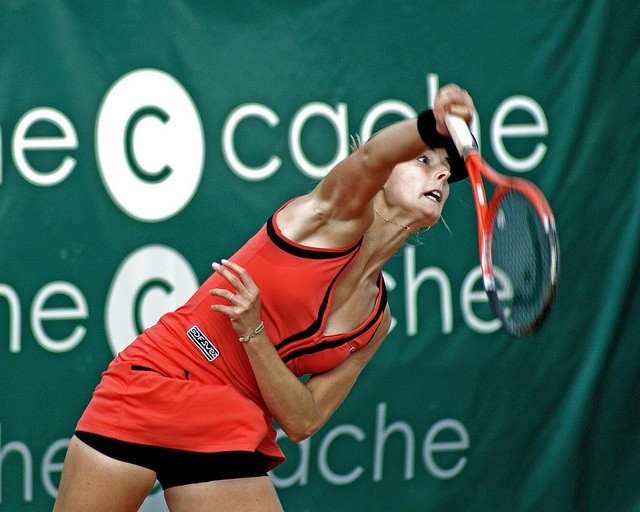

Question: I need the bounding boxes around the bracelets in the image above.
[238,321,266,342]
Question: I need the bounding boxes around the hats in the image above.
[443,131,478,185]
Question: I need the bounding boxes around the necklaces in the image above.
[371,209,413,232]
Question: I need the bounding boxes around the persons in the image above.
[49,83,474,512]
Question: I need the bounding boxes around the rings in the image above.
[229,294,235,301]
